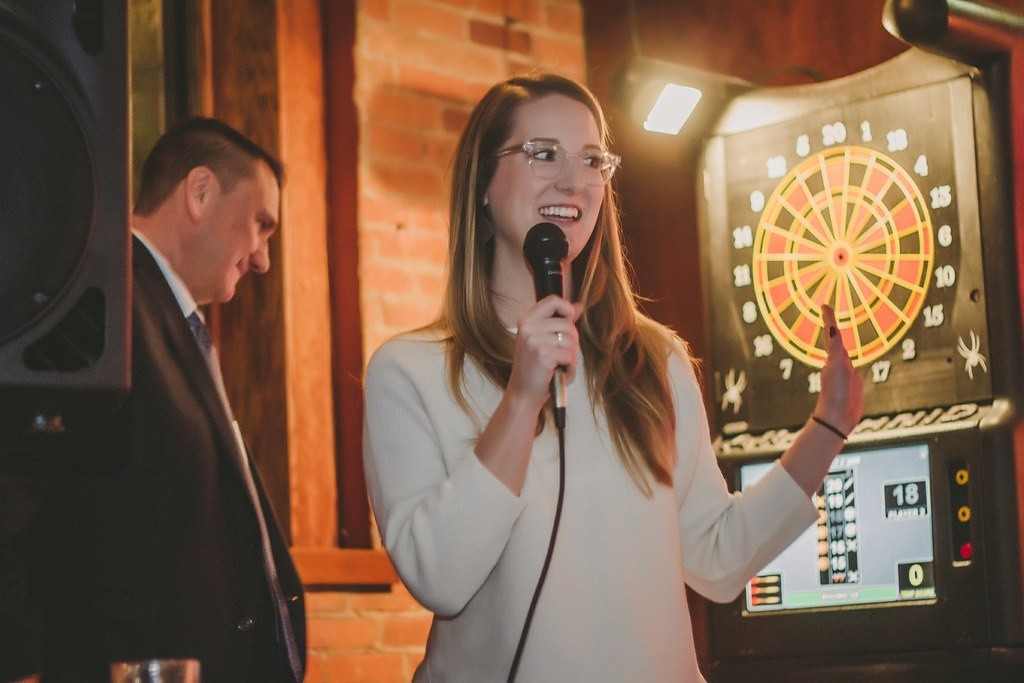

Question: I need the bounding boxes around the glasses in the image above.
[494,140,622,185]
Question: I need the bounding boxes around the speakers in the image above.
[0,0,133,428]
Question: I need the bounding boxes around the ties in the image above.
[187,310,303,683]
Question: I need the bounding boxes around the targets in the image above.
[701,73,996,439]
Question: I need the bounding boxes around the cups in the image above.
[111,658,201,683]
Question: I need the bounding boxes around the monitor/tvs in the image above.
[739,441,936,613]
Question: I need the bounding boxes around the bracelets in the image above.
[809,410,850,446]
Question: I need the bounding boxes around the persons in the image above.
[0,116,308,683]
[363,74,864,683]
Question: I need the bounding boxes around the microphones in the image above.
[524,222,569,428]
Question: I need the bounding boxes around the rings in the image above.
[553,331,566,348]
